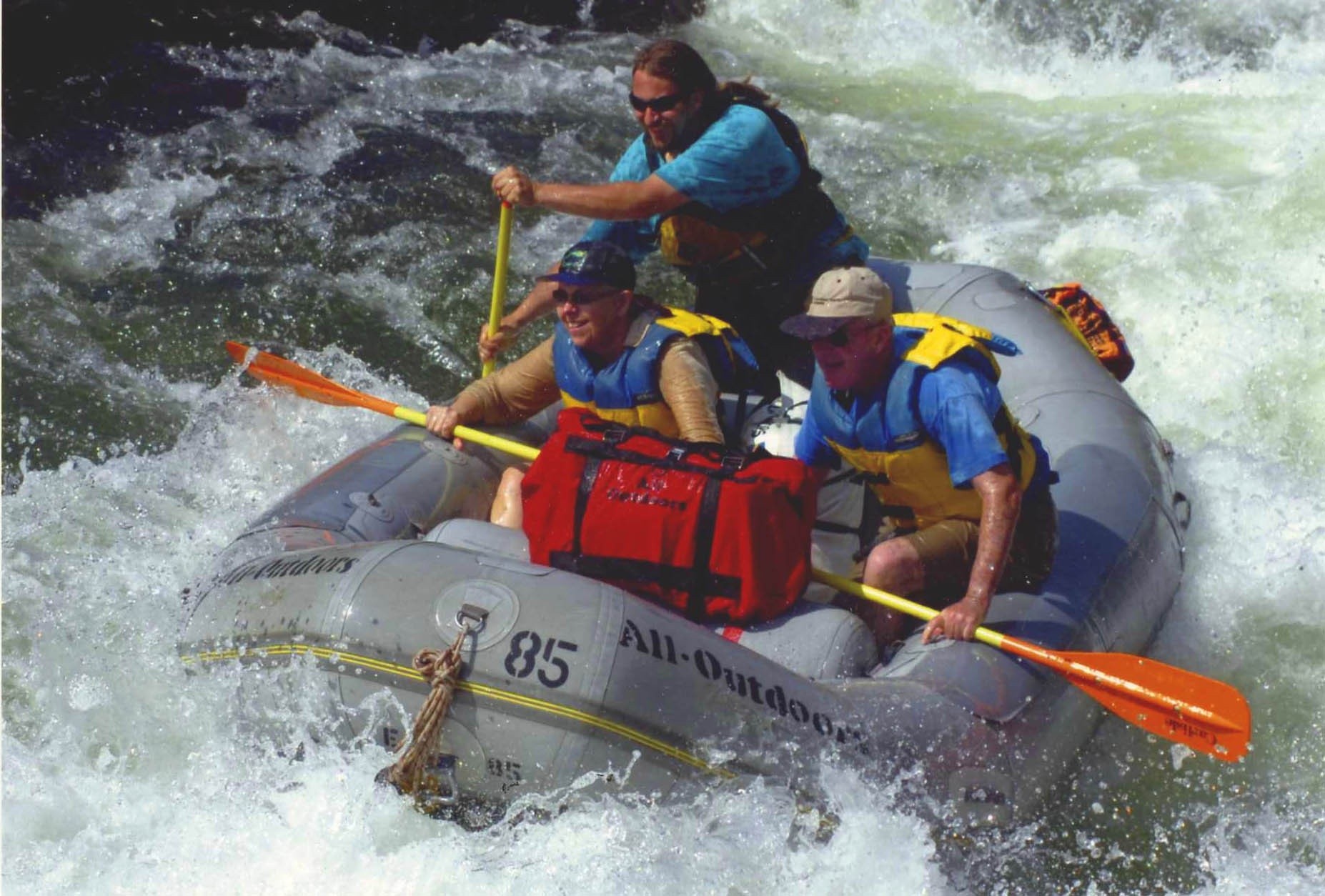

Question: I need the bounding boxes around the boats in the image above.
[174,257,1190,864]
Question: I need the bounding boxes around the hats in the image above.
[534,240,637,289]
[779,266,893,338]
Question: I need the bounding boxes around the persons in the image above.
[426,243,760,530]
[479,39,870,394]
[780,266,1061,646]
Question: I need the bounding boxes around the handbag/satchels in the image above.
[521,407,818,629]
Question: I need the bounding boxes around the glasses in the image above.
[827,319,892,346]
[552,289,622,304]
[628,89,693,113]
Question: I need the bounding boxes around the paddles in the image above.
[224,339,1250,763]
[482,201,513,376]
